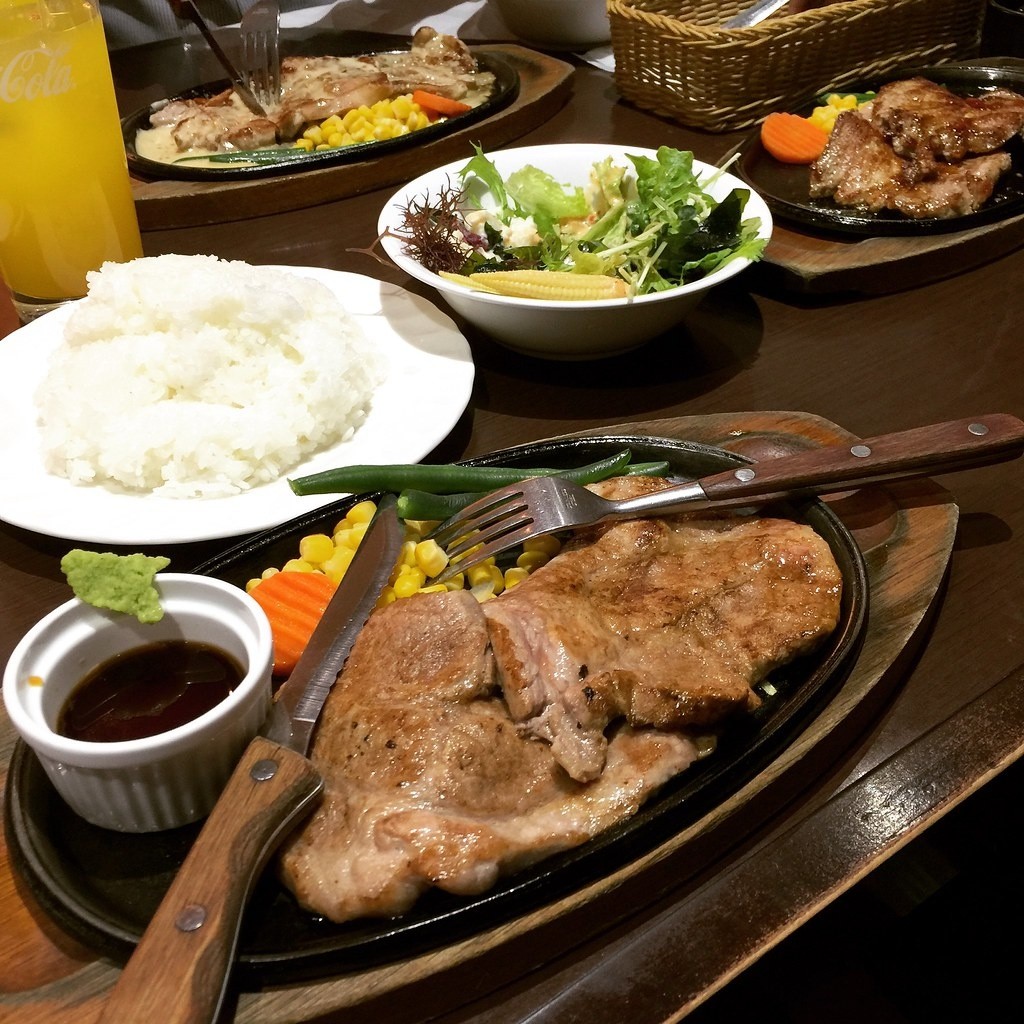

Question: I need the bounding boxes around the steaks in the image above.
[147,26,478,156]
[477,475,844,787]
[808,79,1024,222]
[274,587,700,922]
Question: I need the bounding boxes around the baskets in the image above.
[608,0,985,131]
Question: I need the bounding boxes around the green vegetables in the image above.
[459,138,768,297]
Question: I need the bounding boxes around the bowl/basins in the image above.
[378,142,773,358]
[3,573,273,834]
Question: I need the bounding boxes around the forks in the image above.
[413,411,1024,592]
[240,0,282,102]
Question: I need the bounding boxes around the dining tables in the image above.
[2,24,1024,1024]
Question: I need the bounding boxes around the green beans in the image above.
[287,448,673,524]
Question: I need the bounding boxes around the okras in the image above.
[168,136,387,164]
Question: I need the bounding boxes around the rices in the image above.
[35,250,372,502]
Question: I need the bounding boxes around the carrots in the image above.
[760,112,829,164]
[413,90,471,117]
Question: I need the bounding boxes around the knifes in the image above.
[93,491,407,1024]
[179,0,266,118]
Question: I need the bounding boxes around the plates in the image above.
[734,61,1024,231]
[2,265,476,541]
[119,48,520,184]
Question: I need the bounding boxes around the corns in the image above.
[436,270,632,302]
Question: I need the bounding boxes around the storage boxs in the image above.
[606,2,984,134]
